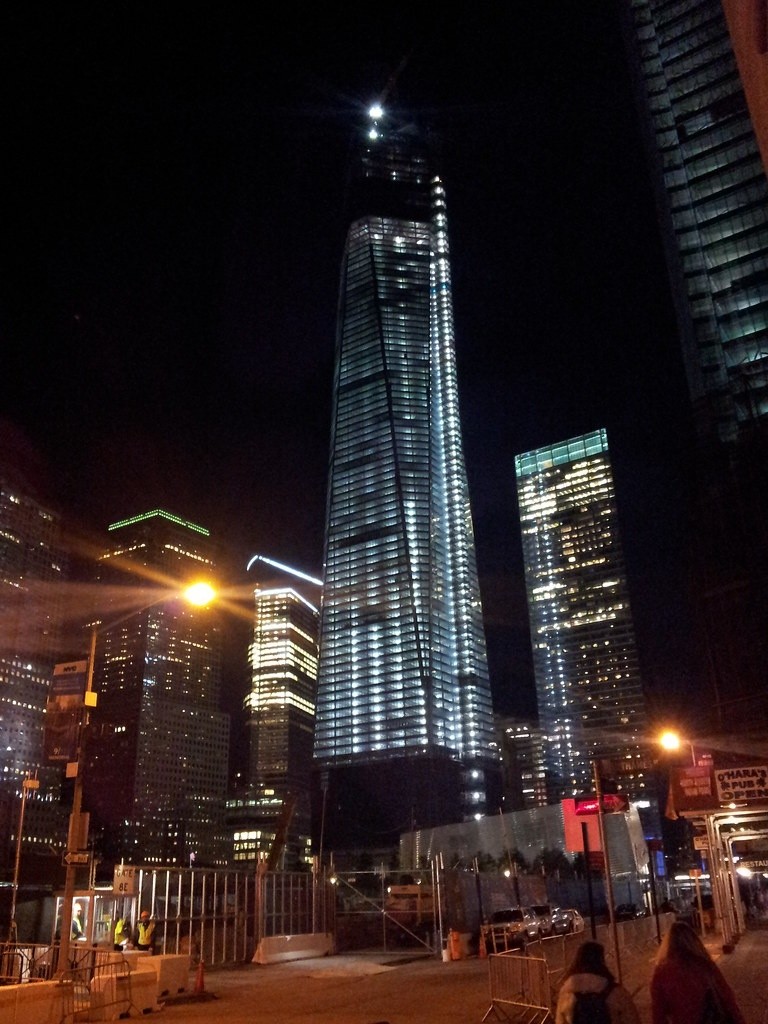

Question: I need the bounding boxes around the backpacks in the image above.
[569,983,616,1024]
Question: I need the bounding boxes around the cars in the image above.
[530,904,585,937]
[614,903,648,922]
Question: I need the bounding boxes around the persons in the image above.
[134,911,156,950]
[70,903,86,940]
[659,897,680,914]
[742,887,768,920]
[114,913,129,951]
[650,920,746,1024]
[555,941,641,1024]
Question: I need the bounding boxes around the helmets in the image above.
[141,911,149,917]
[74,903,81,910]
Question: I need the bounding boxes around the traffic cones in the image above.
[476,935,489,959]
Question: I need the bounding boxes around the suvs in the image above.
[482,906,542,949]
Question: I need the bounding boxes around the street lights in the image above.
[51,581,217,980]
[661,731,697,767]
